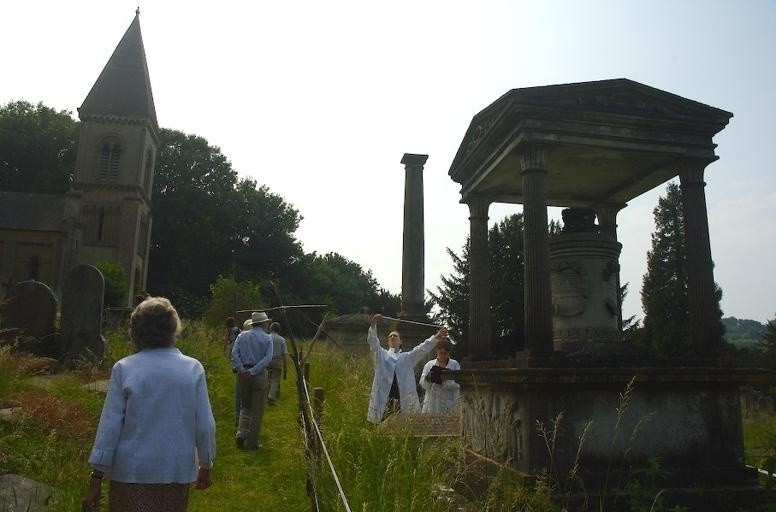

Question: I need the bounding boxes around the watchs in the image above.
[91,472,104,481]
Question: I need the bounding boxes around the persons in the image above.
[81,297,217,512]
[223,312,289,449]
[367,314,460,425]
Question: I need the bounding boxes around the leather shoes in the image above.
[236,437,262,449]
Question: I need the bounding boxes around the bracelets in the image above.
[198,467,208,472]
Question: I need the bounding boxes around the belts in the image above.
[243,364,254,368]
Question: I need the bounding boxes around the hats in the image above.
[243,312,272,326]
